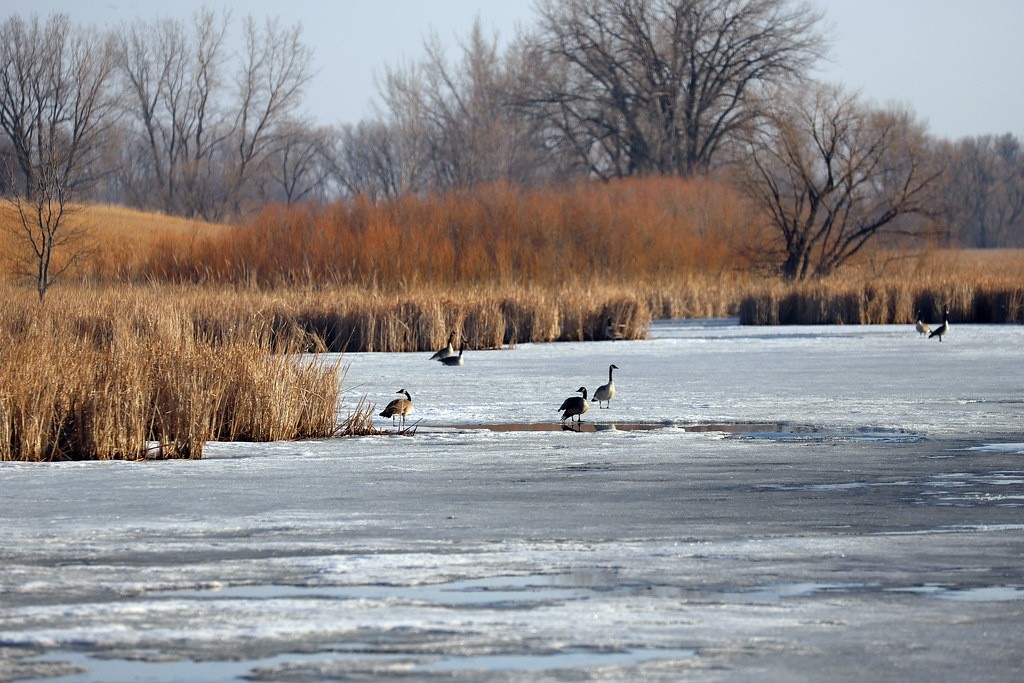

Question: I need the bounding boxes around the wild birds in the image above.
[591,364,619,409]
[556,386,590,423]
[378,388,412,426]
[914,309,950,343]
[429,331,471,367]
